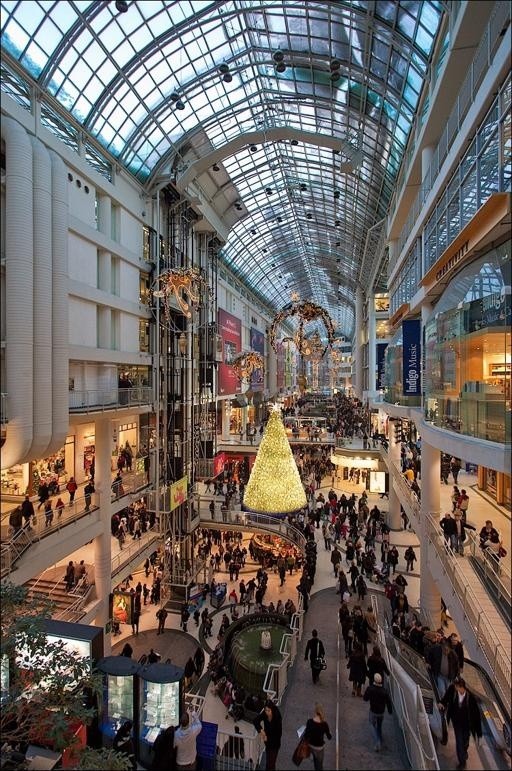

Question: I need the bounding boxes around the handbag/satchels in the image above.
[499,547,508,558]
[295,739,311,759]
[312,656,328,671]
[32,514,38,526]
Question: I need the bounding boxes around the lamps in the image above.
[172,88,186,112]
[235,203,243,212]
[272,263,276,268]
[336,241,341,248]
[233,352,266,404]
[307,213,312,219]
[335,220,341,228]
[274,49,288,74]
[337,259,341,264]
[277,217,283,223]
[334,189,341,200]
[220,60,233,83]
[278,274,283,280]
[283,286,288,289]
[212,162,221,172]
[300,183,308,192]
[265,186,273,196]
[336,270,342,332]
[250,228,258,236]
[330,57,343,83]
[149,209,212,355]
[291,140,298,146]
[332,149,340,156]
[270,299,336,396]
[250,143,259,153]
[261,248,268,254]
[116,1,130,17]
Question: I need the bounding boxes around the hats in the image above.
[451,676,465,688]
[372,672,383,688]
